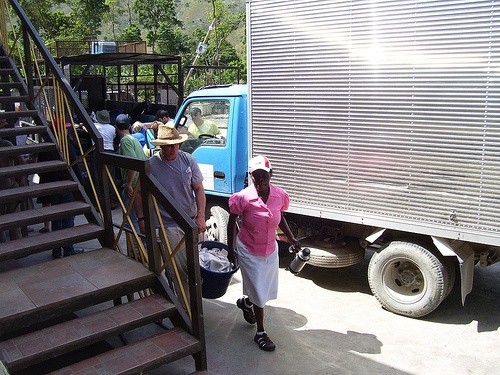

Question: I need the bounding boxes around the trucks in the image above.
[145,0,500,318]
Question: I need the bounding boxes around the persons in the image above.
[15,117,27,158]
[130,121,146,147]
[17,176,34,232]
[0,118,25,243]
[37,119,84,259]
[133,126,206,312]
[188,107,221,139]
[227,155,301,351]
[90,110,118,212]
[114,114,147,261]
[142,109,174,129]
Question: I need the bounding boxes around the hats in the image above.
[151,125,188,145]
[96,110,111,124]
[49,119,71,130]
[188,107,201,114]
[248,156,272,175]
[116,114,129,125]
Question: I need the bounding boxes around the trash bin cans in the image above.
[204,103,212,114]
[192,105,203,116]
[203,104,208,115]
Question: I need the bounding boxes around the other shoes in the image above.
[52,250,63,258]
[64,247,84,257]
[39,228,50,234]
[111,203,117,209]
[26,226,34,232]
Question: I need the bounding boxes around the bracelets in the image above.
[138,217,144,222]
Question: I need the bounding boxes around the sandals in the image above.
[254,333,275,351]
[237,299,256,324]
